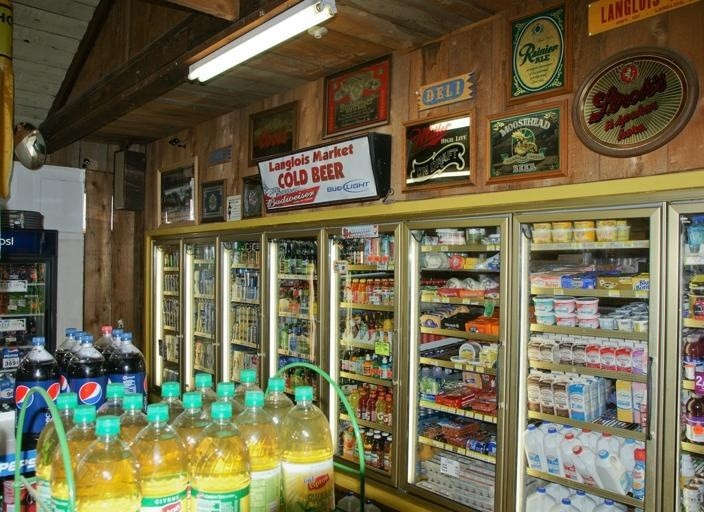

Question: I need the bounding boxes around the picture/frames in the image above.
[506,0,573,107]
[199,178,226,223]
[321,53,392,140]
[572,46,699,158]
[485,99,568,185]
[248,100,301,167]
[155,156,198,230]
[401,107,478,194]
[242,173,266,218]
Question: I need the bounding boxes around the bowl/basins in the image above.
[3,210,44,228]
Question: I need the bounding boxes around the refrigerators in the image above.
[0,230,58,408]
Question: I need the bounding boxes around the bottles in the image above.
[685,330,704,380]
[522,420,645,512]
[680,454,695,490]
[684,391,704,446]
[355,499,381,512]
[16,325,146,477]
[33,368,334,511]
[337,492,360,512]
[688,215,704,256]
[688,274,704,322]
[419,365,461,404]
[339,235,394,473]
[159,240,260,397]
[279,238,320,401]
[0,262,45,348]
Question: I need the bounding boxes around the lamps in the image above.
[187,0,337,84]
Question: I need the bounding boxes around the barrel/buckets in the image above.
[523,420,646,512]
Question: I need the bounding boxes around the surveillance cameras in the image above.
[170,138,185,148]
[83,160,91,167]
